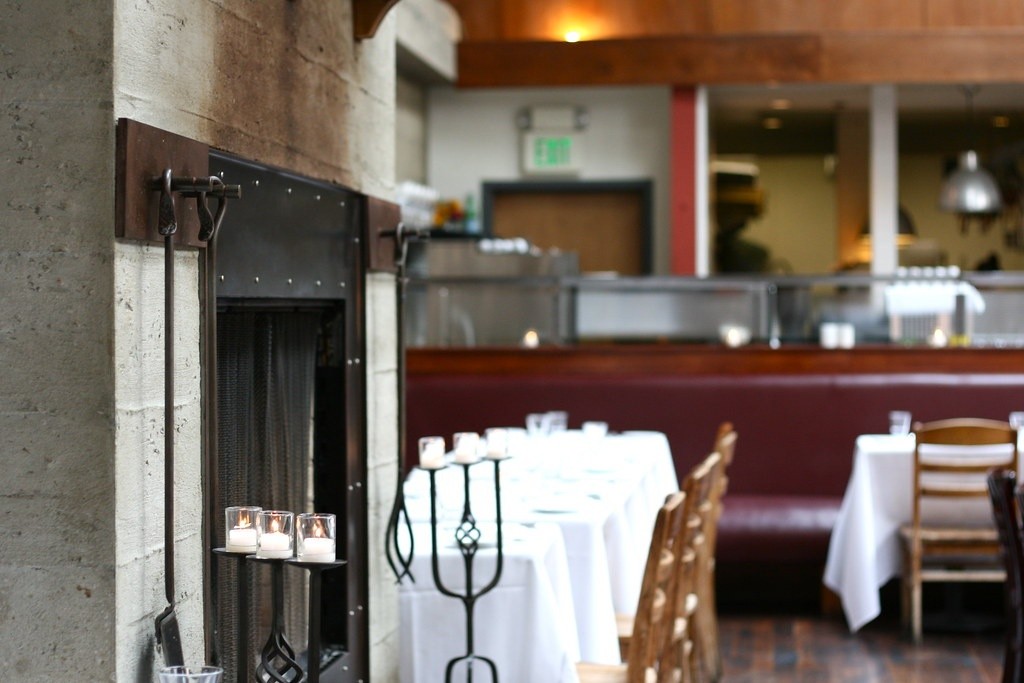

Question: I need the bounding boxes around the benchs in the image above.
[396,367,1024,577]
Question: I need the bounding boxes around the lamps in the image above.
[936,83,1006,216]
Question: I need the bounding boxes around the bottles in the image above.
[462,194,478,232]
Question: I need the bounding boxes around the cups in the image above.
[256,510,295,559]
[888,410,912,437]
[225,506,262,553]
[523,408,609,446]
[297,512,336,564]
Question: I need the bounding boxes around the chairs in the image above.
[894,418,1024,648]
[978,461,1024,683]
[576,423,740,683]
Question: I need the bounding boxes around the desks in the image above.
[820,433,1024,634]
[379,426,697,680]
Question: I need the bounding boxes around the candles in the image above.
[423,438,443,462]
[302,527,334,555]
[260,519,290,551]
[228,517,257,546]
[486,428,506,455]
[456,432,476,456]
